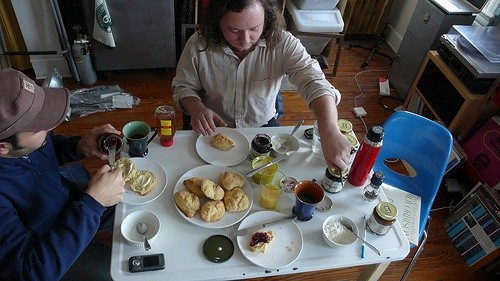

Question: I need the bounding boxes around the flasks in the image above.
[347,126,385,186]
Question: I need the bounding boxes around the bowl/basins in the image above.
[271,133,300,160]
[121,210,162,247]
[321,215,359,248]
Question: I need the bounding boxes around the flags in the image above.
[92,0,115,48]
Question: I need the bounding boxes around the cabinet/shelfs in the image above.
[195,0,355,77]
[401,51,500,143]
[390,0,482,101]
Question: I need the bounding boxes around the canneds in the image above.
[99,134,123,154]
[337,119,352,134]
[251,133,272,161]
[322,166,349,194]
[343,132,358,164]
[366,202,397,237]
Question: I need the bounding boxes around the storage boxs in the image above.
[284,0,344,55]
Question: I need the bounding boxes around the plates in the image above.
[236,211,304,270]
[111,157,169,205]
[195,126,250,167]
[172,164,254,229]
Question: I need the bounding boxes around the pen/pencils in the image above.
[361,214,366,258]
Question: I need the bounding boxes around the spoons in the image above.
[136,222,152,252]
[339,220,382,256]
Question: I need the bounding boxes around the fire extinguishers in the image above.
[71,24,97,86]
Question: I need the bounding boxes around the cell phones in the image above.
[128,253,165,273]
[304,128,313,139]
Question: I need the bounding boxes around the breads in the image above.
[174,171,249,222]
[129,170,157,196]
[110,158,136,181]
[250,230,275,252]
[211,133,236,151]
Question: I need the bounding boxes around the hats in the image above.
[0,68,70,140]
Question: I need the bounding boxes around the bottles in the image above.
[154,105,177,147]
[248,132,272,162]
[362,171,384,201]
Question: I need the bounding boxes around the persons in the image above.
[171,0,352,171]
[0,67,126,281]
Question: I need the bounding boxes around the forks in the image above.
[276,119,304,153]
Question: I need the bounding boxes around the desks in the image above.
[110,126,410,281]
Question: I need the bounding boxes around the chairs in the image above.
[373,111,454,281]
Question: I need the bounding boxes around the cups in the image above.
[260,169,287,210]
[98,134,124,155]
[292,179,325,221]
[121,120,152,158]
[311,119,323,154]
[252,155,279,184]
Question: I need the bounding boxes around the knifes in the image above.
[244,154,287,177]
[230,214,297,238]
